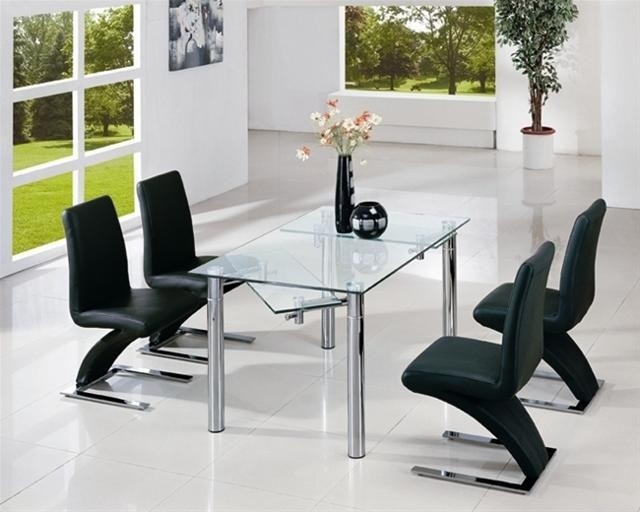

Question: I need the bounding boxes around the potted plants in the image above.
[493,0,579,171]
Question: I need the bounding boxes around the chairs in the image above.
[401,240,557,495]
[473,199,606,416]
[136,170,256,364]
[59,195,203,411]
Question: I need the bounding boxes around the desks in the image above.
[187,204,471,459]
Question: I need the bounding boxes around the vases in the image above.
[335,155,355,233]
[349,202,388,239]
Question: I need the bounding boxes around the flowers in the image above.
[295,98,383,168]
